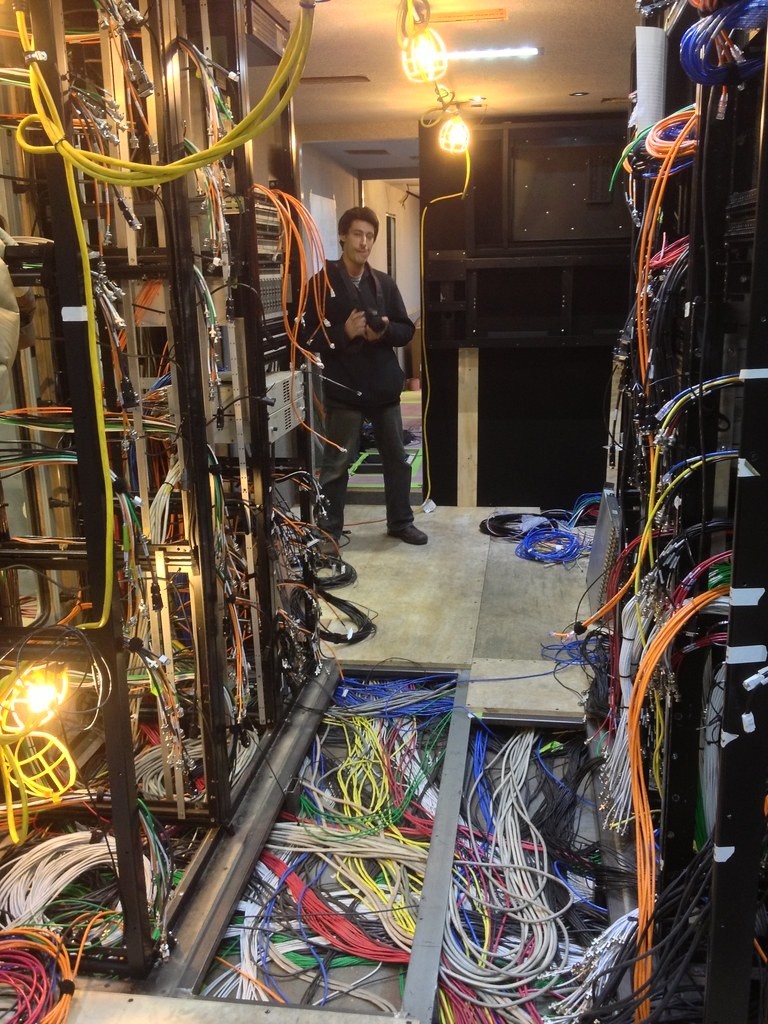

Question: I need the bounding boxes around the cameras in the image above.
[358,308,385,332]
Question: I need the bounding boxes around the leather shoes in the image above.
[386,525,428,546]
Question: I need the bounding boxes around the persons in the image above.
[303,203,428,557]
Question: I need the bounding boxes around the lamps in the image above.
[437,104,470,154]
[402,26,449,83]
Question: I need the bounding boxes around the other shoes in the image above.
[321,538,339,557]
